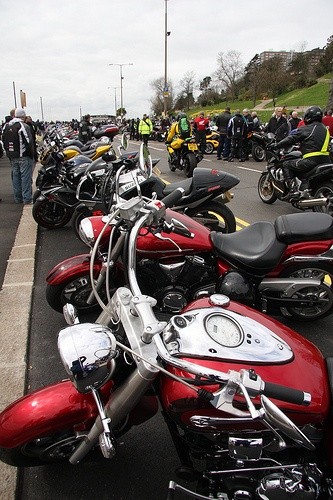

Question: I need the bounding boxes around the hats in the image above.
[14,107,26,117]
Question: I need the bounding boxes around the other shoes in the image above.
[228,157,234,162]
[238,158,245,163]
[218,157,228,161]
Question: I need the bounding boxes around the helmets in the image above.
[303,106,323,125]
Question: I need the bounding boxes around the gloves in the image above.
[266,143,278,151]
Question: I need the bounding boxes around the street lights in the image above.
[108,63,133,122]
[107,86,122,117]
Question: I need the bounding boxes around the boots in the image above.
[281,178,301,201]
[170,153,179,164]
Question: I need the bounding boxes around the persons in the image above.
[165,112,201,163]
[138,113,153,149]
[193,112,209,158]
[269,107,330,200]
[251,111,260,128]
[216,107,231,160]
[0,107,333,197]
[78,114,92,145]
[243,108,254,161]
[227,109,246,162]
[1,108,36,205]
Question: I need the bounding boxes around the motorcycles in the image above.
[0,170,333,500]
[44,154,333,323]
[0,113,333,250]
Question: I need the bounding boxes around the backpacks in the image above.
[177,117,192,141]
